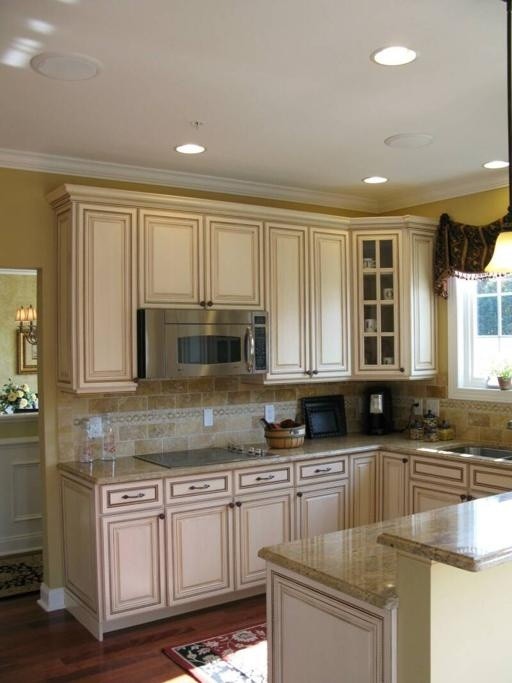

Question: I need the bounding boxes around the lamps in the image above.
[16,304,38,346]
[484,0,512,274]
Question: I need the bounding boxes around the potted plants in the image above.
[497,365,511,389]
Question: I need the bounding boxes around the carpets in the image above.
[0,551,43,600]
[162,621,267,683]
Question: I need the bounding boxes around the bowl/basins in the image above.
[264,422,306,448]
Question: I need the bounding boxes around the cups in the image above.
[382,355,394,363]
[383,287,393,298]
[363,256,376,268]
[364,318,376,332]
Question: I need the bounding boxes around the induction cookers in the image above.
[131,444,278,468]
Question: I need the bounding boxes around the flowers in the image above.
[0,378,38,413]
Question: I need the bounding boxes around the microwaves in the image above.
[142,306,272,380]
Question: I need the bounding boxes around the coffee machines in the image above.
[366,386,394,434]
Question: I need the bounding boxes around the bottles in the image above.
[80,418,94,462]
[98,411,118,460]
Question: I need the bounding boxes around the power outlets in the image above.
[414,399,423,417]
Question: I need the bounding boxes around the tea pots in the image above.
[408,408,454,441]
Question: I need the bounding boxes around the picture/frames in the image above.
[304,403,345,439]
[17,331,39,375]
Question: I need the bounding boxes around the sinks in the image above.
[436,440,511,460]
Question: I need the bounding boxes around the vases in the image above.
[3,403,15,412]
